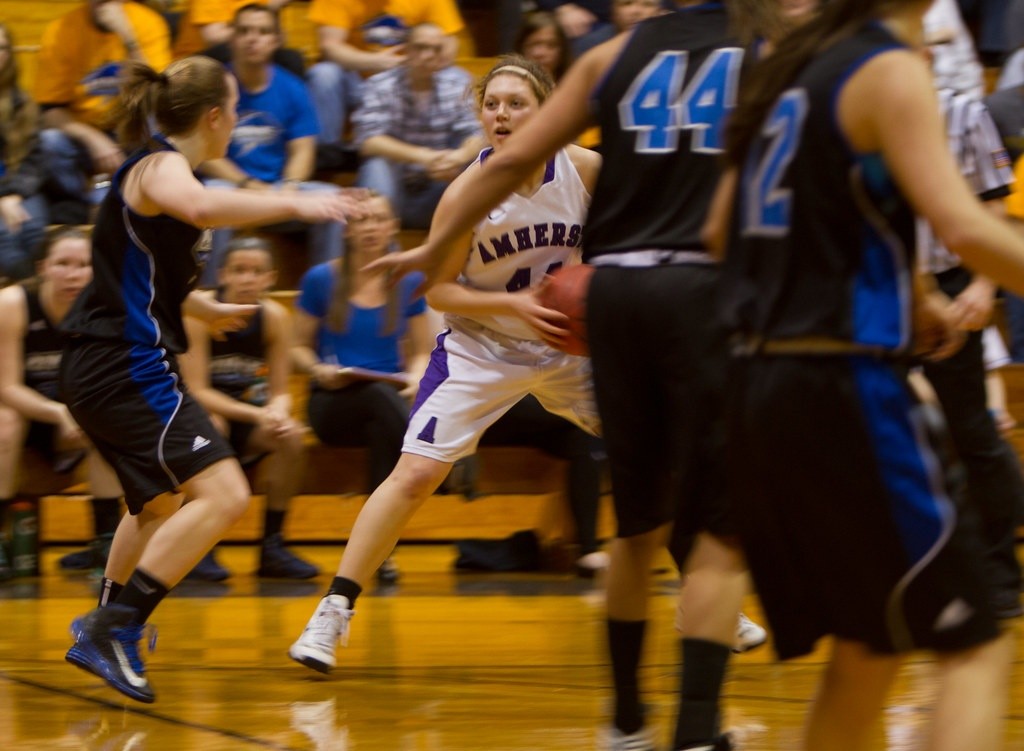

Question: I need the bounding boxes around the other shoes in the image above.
[59,541,112,568]
[378,560,397,580]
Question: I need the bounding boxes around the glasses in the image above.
[235,26,278,36]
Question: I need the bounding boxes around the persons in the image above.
[66,55,372,702]
[701,0,1024,750]
[0,0,1024,615]
[360,0,783,751]
[286,59,766,674]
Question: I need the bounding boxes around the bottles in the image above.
[87,173,111,224]
[10,501,39,577]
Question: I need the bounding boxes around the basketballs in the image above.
[539,263,592,357]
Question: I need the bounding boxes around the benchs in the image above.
[38,176,617,542]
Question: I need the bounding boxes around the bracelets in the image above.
[240,176,255,186]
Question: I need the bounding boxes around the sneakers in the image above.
[65,627,154,703]
[673,592,766,652]
[259,547,317,579]
[72,607,113,639]
[184,551,229,582]
[288,595,355,675]
